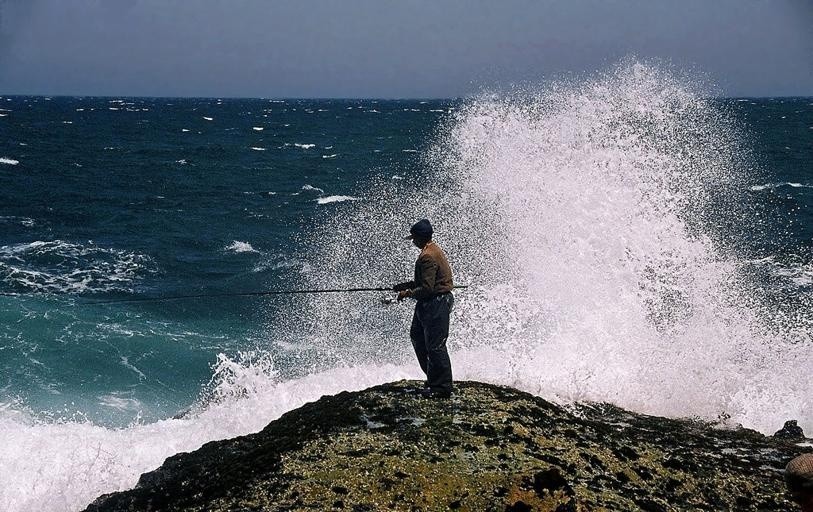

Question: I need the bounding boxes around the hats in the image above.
[405,219,434,240]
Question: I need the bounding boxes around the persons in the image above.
[393,218,454,397]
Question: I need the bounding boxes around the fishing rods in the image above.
[78,285,468,305]
